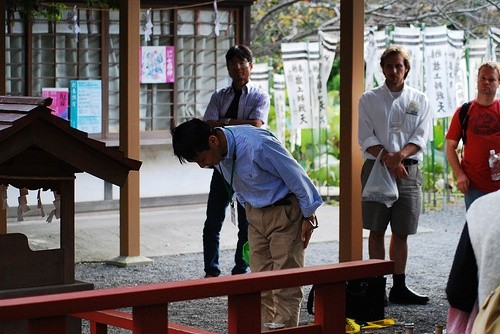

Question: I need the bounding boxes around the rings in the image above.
[303,215,318,229]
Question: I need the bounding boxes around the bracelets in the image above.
[225,118,231,126]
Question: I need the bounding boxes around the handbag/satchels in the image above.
[361,148,400,208]
[307,278,386,322]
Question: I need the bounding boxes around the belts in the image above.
[262,193,294,208]
[401,159,418,166]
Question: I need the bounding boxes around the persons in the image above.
[445,62,500,334]
[172,118,322,331]
[202,44,271,278]
[357,46,431,304]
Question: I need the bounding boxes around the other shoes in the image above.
[389,286,430,305]
